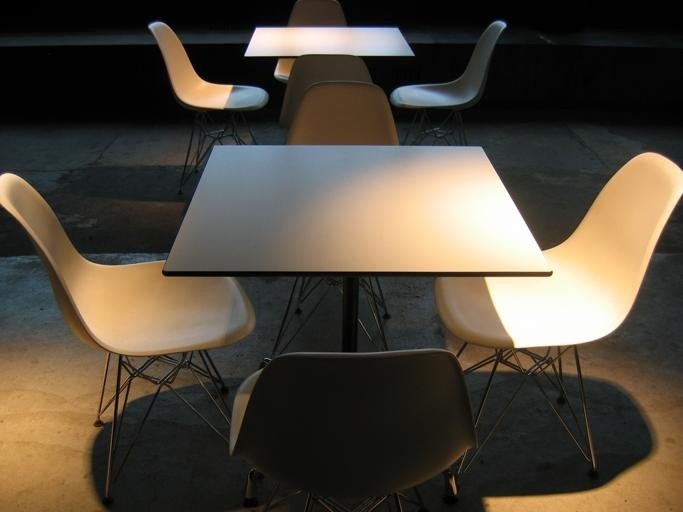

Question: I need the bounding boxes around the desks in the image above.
[243,26,415,59]
[162,145,553,351]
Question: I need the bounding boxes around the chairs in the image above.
[435,152,683,473]
[389,21,507,146]
[279,54,374,127]
[149,21,270,195]
[230,349,477,510]
[273,0,350,81]
[270,81,400,355]
[0,171,257,500]
